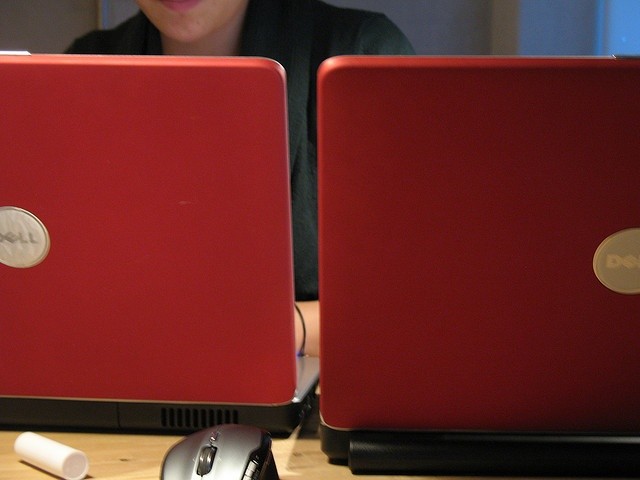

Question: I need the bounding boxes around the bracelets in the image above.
[293,302,306,358]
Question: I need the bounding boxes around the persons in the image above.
[62,0,418,359]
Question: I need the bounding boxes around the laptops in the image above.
[1,53,316,439]
[315,54,640,480]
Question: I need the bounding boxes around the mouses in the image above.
[160,423,272,480]
